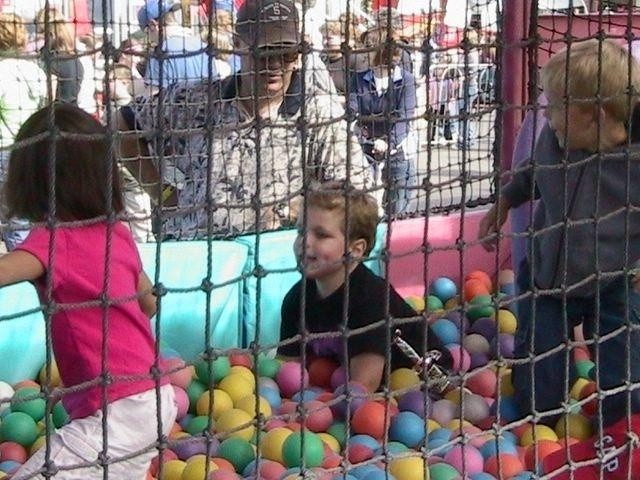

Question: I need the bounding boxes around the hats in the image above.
[136,0,183,33]
[233,0,304,50]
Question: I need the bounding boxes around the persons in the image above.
[1,0,502,241]
[479,40,640,436]
[0,97,177,480]
[273,180,455,394]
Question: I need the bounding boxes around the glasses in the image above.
[233,33,299,66]
